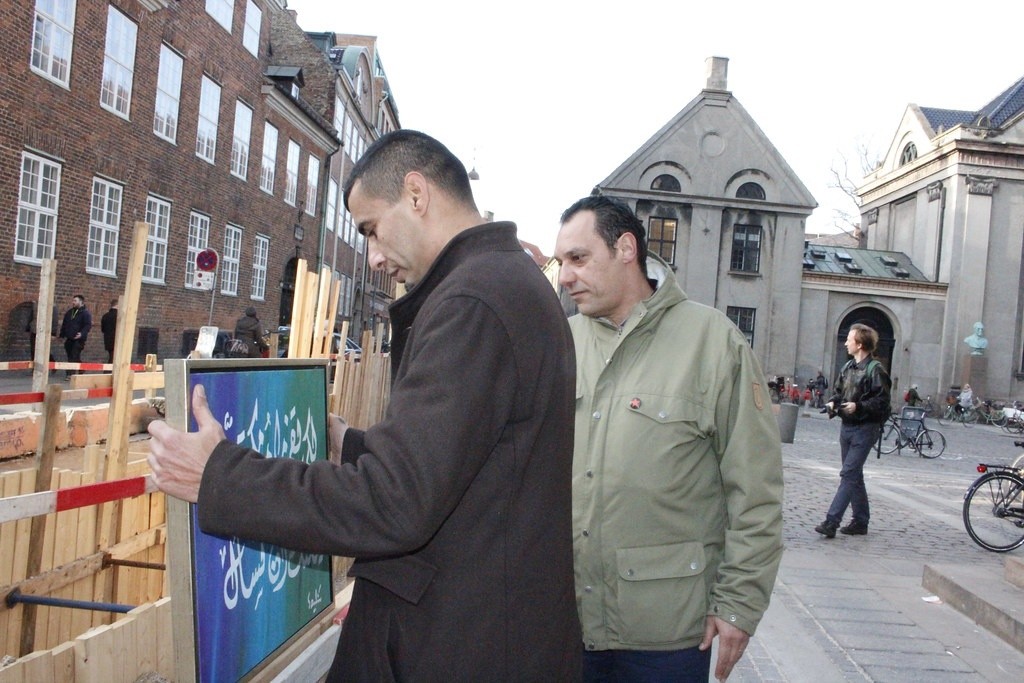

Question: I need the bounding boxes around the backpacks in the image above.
[823,378,828,388]
[840,359,891,424]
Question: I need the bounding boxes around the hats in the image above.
[911,383,917,388]
[245,307,255,316]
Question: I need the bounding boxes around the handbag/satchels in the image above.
[903,391,909,400]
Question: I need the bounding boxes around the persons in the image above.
[814,323,893,538]
[809,370,828,408]
[58,294,92,381]
[234,306,270,358]
[101,299,118,373]
[963,322,988,350]
[955,383,973,421]
[554,197,784,683]
[24,296,58,376]
[904,383,923,406]
[146,129,584,683]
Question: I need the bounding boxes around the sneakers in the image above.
[841,520,868,534]
[816,520,839,538]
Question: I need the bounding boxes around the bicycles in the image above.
[974,407,1024,438]
[960,458,1024,549]
[938,404,979,428]
[805,382,825,408]
[766,384,783,407]
[869,408,946,460]
[786,378,801,405]
